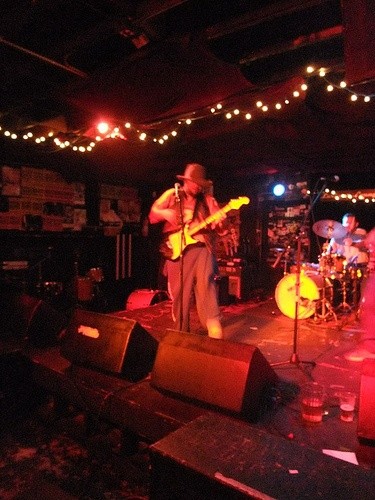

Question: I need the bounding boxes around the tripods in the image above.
[270,185,361,379]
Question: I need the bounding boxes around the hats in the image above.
[176,163,210,188]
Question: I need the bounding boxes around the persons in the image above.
[319,213,370,313]
[148,164,228,338]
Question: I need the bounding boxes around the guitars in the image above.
[158,195,251,261]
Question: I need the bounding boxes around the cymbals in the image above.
[312,220,347,239]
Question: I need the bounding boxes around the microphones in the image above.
[174,183,181,198]
[321,175,340,182]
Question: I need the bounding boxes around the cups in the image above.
[340,393,356,421]
[302,381,326,423]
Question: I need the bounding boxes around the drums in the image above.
[318,254,347,275]
[275,271,334,320]
[344,266,369,281]
[288,260,318,274]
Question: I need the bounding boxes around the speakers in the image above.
[213,275,249,306]
[8,293,68,348]
[356,375,375,448]
[148,326,280,420]
[69,309,159,384]
